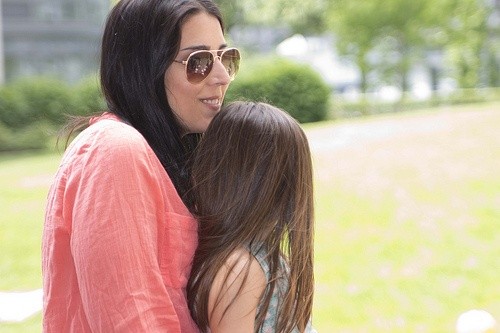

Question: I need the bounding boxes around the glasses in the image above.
[170,48,242,84]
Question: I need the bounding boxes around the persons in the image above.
[40,0,241,333]
[185,99,316,333]
